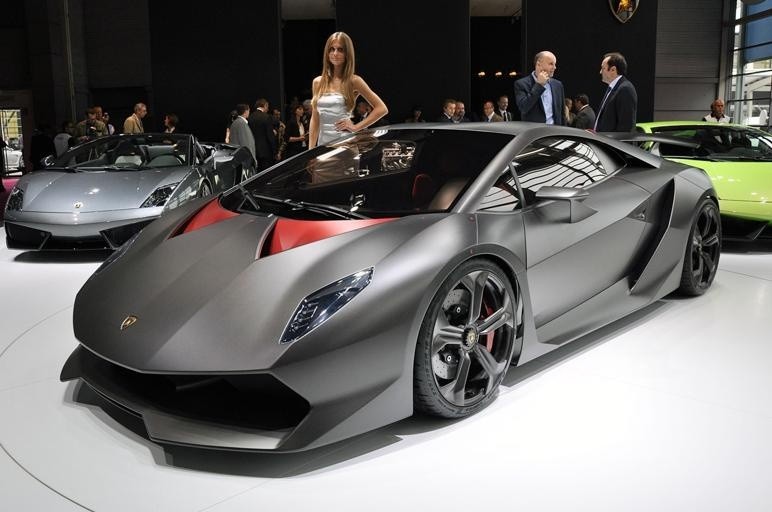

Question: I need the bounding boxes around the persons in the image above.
[306,31,389,184]
[565,97,577,118]
[123,103,147,133]
[437,99,456,123]
[303,99,312,116]
[75,108,105,139]
[52,121,75,158]
[94,106,109,125]
[513,51,566,126]
[163,114,180,134]
[283,104,307,155]
[270,108,286,161]
[482,102,504,123]
[230,104,258,170]
[407,108,425,123]
[495,95,512,122]
[703,100,732,123]
[250,99,276,166]
[451,101,469,123]
[592,52,638,133]
[571,94,596,131]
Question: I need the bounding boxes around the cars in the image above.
[3,145,25,172]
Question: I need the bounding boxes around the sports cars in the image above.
[3,132,218,254]
[633,119,772,231]
[59,120,725,456]
[199,140,257,194]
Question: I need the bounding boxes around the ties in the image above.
[504,112,506,121]
[594,87,611,124]
